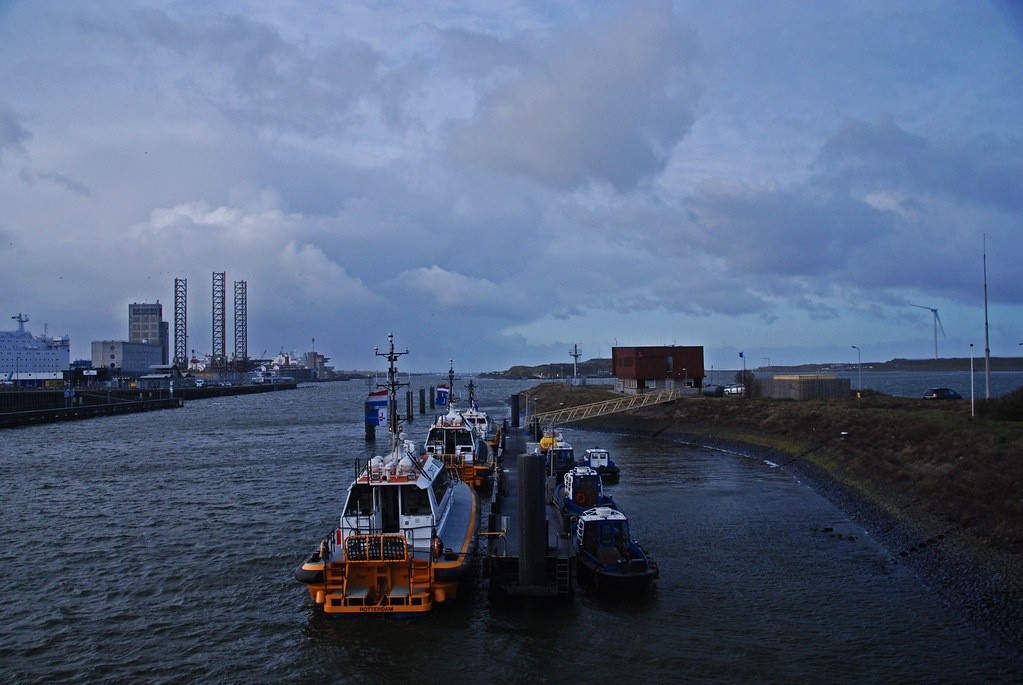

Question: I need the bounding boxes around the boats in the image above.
[422,357,495,494]
[553,465,625,526]
[295,331,483,618]
[456,379,501,454]
[579,445,620,483]
[530,416,577,472]
[571,505,657,607]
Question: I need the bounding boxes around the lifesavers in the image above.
[434,538,439,555]
[576,493,585,503]
[320,541,328,558]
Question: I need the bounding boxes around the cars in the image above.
[218,382,231,386]
[723,383,746,395]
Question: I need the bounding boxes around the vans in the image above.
[922,388,963,400]
[196,379,204,387]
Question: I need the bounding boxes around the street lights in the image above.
[852,345,862,391]
